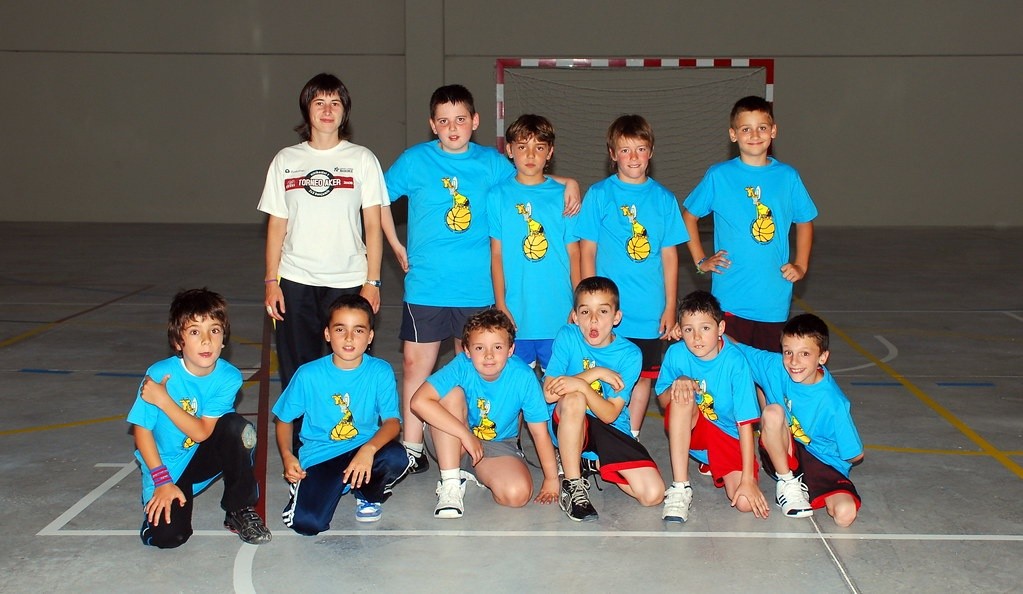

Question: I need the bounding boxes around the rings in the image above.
[266,306,273,315]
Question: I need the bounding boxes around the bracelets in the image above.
[697,258,708,274]
[264,279,278,284]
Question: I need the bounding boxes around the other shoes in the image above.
[699,461,710,475]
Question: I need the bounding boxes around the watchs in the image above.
[365,280,381,287]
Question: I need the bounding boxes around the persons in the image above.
[655,293,770,524]
[682,96,818,435]
[271,297,411,537]
[667,313,864,528]
[488,115,580,451]
[572,117,691,442]
[256,73,391,458]
[127,289,271,549]
[380,84,581,474]
[541,277,666,523]
[410,309,561,518]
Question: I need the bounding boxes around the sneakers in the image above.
[404,445,429,474]
[662,483,693,523]
[556,449,565,474]
[223,506,272,544]
[356,498,383,522]
[580,457,603,491]
[775,473,813,519]
[342,484,351,494]
[558,477,599,522]
[434,477,467,518]
